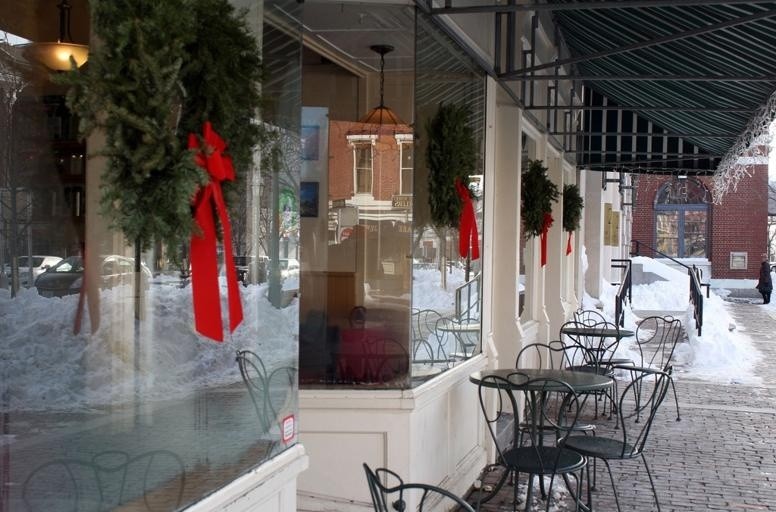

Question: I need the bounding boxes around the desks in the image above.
[564,328,635,411]
[471,367,612,502]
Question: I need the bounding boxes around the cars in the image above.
[4,255,72,288]
[231,255,268,286]
[35,254,153,297]
[268,257,299,278]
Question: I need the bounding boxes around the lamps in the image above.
[21,4,89,76]
[346,44,414,147]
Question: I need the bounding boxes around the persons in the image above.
[755,253,773,304]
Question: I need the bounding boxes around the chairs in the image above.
[514,340,591,487]
[553,372,673,511]
[560,320,620,424]
[477,372,589,509]
[21,448,187,511]
[617,314,681,422]
[574,312,618,413]
[233,349,299,459]
[365,462,474,509]
